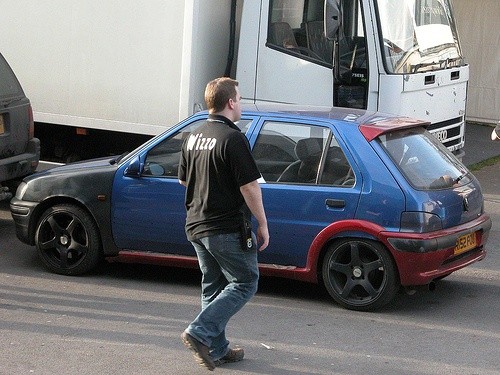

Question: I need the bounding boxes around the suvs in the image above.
[0,53,40,198]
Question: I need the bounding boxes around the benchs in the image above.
[277,138,353,186]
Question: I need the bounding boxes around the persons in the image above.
[178,78,269,370]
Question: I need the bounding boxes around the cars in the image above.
[10,104,491,313]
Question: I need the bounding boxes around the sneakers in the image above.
[181,332,216,371]
[214,348,244,367]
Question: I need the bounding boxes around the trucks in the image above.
[0,0,469,174]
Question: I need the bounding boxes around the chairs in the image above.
[301,20,351,64]
[269,22,301,52]
